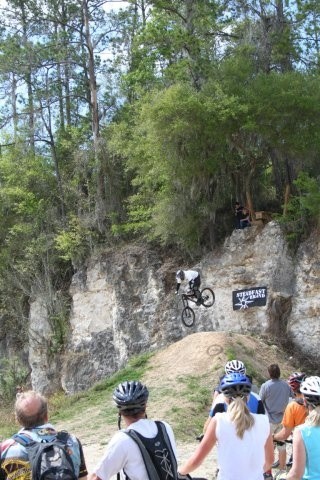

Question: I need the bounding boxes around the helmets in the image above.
[176,270,184,278]
[289,372,305,381]
[219,373,251,390]
[225,360,246,377]
[299,376,320,396]
[112,380,149,410]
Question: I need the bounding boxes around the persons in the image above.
[198,359,266,436]
[88,380,178,480]
[174,269,203,306]
[211,374,225,402]
[0,389,88,480]
[232,202,245,229]
[258,363,295,473]
[271,375,320,480]
[271,371,309,442]
[177,371,274,480]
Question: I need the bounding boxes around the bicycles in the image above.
[192,433,293,480]
[174,286,216,326]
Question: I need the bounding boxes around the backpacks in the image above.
[11,432,78,480]
